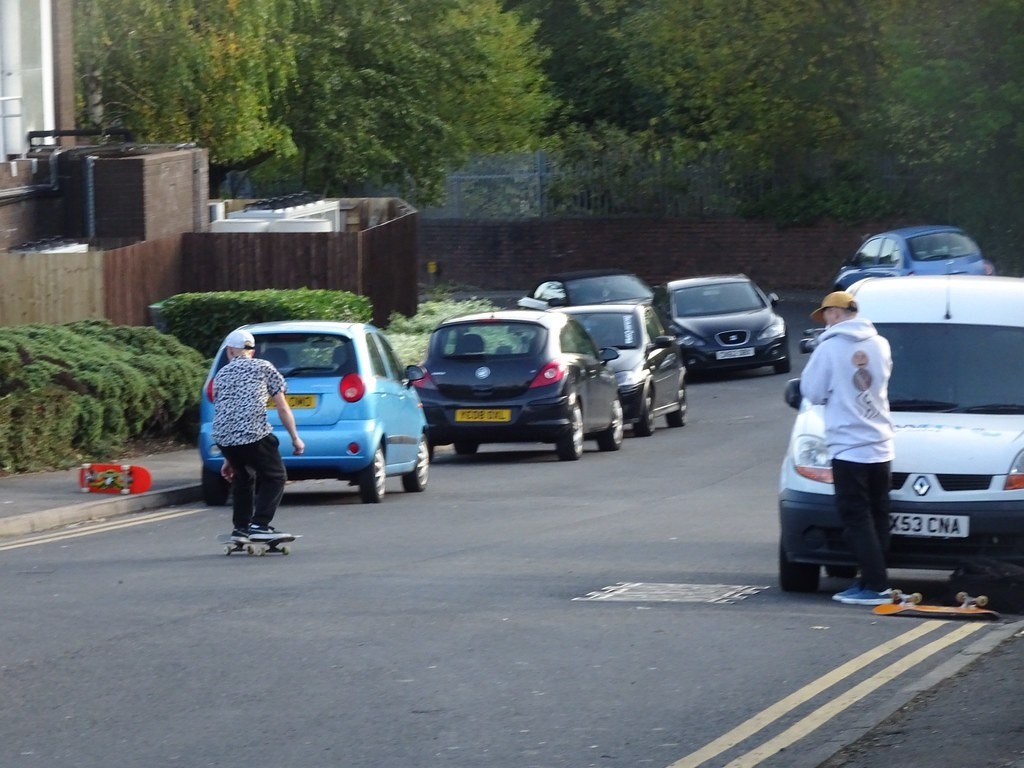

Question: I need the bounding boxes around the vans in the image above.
[775,272,1024,594]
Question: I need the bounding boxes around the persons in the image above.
[213,329,306,545]
[798,290,895,605]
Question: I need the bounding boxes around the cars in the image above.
[832,227,997,295]
[411,310,626,462]
[524,303,690,436]
[199,320,431,503]
[665,273,793,379]
[523,269,661,317]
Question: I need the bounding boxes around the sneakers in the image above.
[231,528,255,543]
[832,587,861,601]
[842,588,894,605]
[247,523,294,542]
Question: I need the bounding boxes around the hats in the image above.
[809,291,858,324]
[220,330,255,349]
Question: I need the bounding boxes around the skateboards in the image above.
[871,588,1004,622]
[78,461,152,494]
[215,533,303,557]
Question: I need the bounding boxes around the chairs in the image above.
[259,348,290,369]
[331,346,346,369]
[457,334,485,354]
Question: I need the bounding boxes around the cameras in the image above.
[799,328,827,353]
